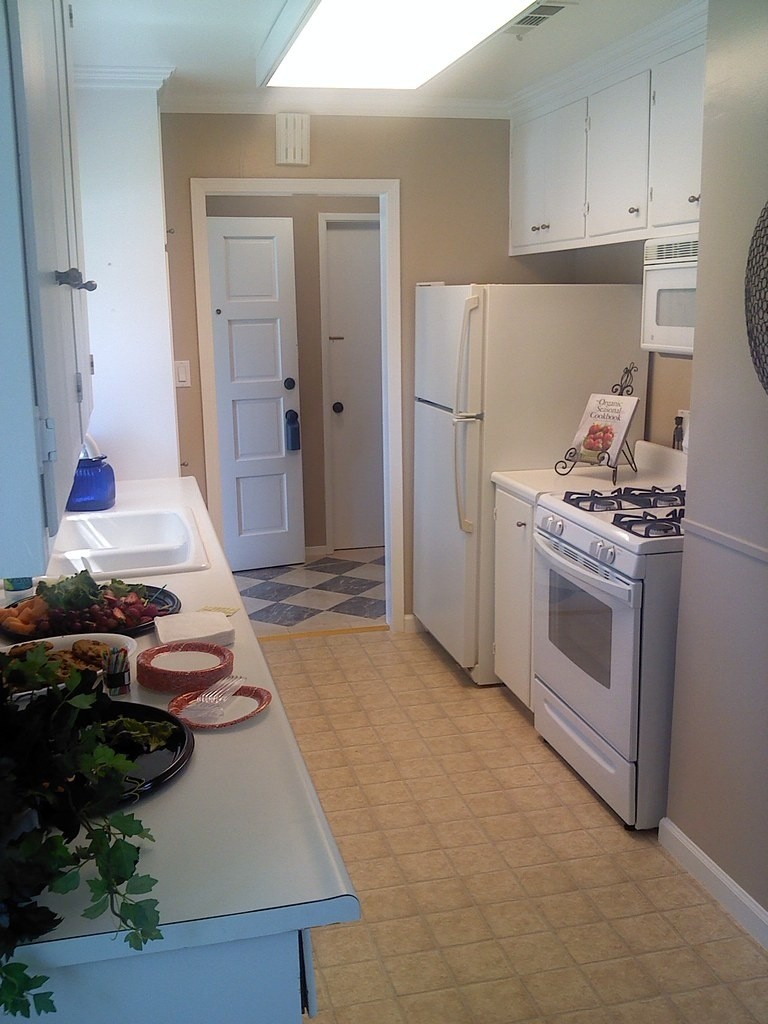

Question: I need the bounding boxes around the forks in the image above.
[177,676,248,725]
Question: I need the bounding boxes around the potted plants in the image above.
[0,637,164,1018]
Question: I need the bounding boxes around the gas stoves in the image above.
[537,484,686,554]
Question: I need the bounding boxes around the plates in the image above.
[136,642,235,693]
[0,584,181,638]
[0,701,195,825]
[0,633,137,701]
[168,686,272,729]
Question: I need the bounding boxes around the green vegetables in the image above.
[31,569,147,611]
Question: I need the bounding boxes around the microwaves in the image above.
[640,234,699,359]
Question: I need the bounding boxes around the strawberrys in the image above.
[582,424,612,451]
[103,591,162,630]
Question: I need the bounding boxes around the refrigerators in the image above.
[411,281,649,687]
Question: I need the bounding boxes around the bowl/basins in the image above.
[0,577,42,608]
[579,436,606,460]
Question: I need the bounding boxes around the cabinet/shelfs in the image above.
[650,45,704,227]
[586,70,650,236]
[8,1,95,536]
[493,488,533,707]
[509,96,586,247]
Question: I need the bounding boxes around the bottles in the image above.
[66,453,116,511]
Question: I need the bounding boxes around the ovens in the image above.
[532,505,683,831]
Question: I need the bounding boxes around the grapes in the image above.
[38,604,113,635]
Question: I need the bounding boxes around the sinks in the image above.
[45,505,207,554]
[44,542,212,581]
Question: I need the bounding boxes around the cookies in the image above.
[0,639,113,694]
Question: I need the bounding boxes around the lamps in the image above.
[275,112,311,167]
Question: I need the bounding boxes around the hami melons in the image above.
[0,595,48,635]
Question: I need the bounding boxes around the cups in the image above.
[101,661,132,701]
